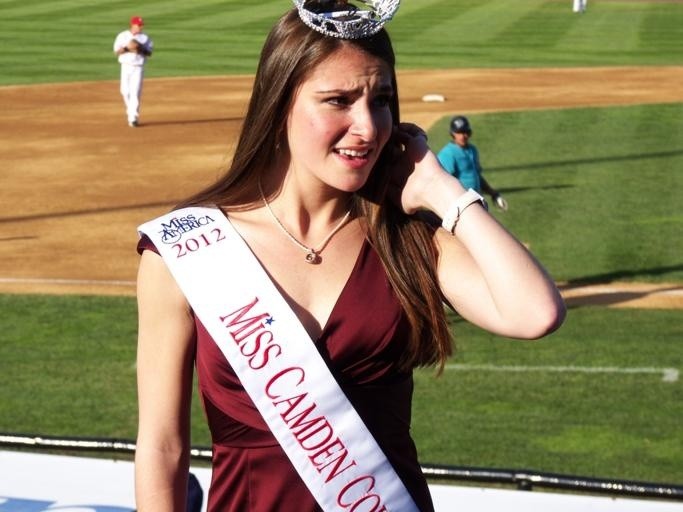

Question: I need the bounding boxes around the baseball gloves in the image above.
[129,40,143,54]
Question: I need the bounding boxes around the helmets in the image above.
[448,115,470,135]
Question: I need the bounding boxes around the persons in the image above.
[111,17,155,130]
[130,1,568,512]
[571,1,588,14]
[434,116,510,211]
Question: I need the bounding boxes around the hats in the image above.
[131,16,144,26]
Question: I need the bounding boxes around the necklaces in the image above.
[255,173,356,267]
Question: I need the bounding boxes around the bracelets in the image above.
[440,187,491,237]
